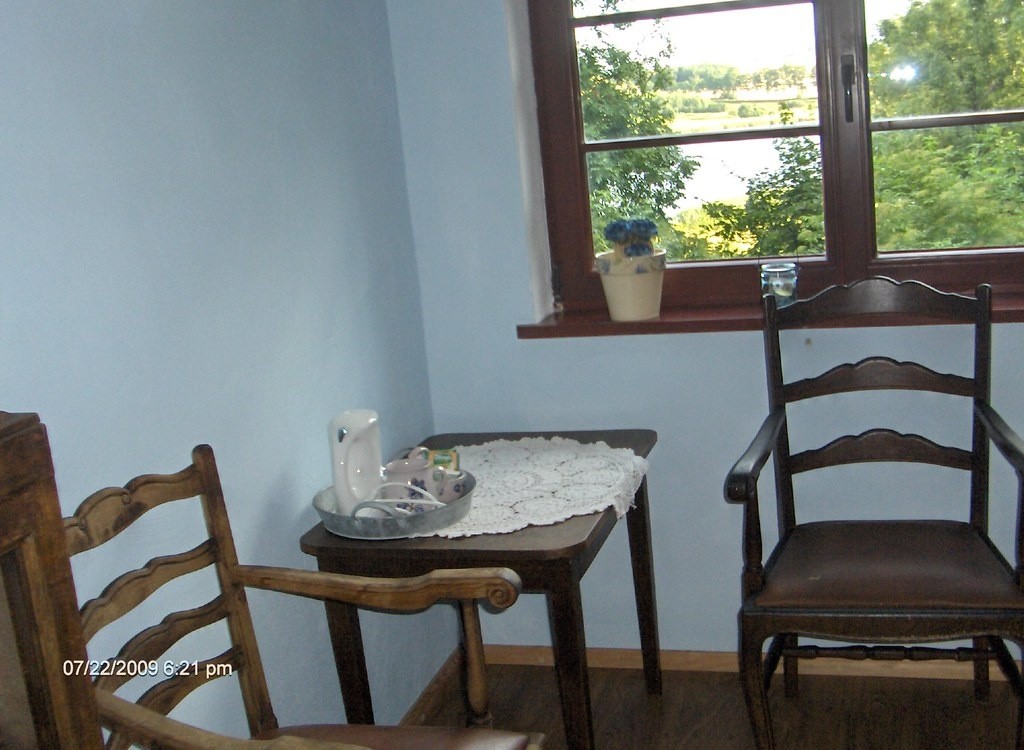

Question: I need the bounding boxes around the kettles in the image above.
[327,409,383,520]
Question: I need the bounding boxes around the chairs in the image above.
[61,443,546,750]
[724,275,1024,750]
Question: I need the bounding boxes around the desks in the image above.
[299,428,663,750]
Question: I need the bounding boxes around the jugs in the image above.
[385,446,438,512]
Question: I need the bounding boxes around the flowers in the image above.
[604,217,658,258]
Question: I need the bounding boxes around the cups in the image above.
[429,462,467,505]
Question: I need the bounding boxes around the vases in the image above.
[595,248,667,321]
